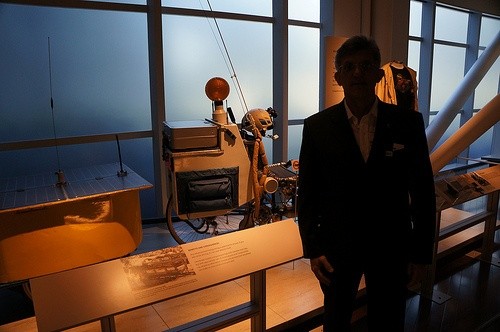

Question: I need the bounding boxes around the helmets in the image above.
[242,108,273,131]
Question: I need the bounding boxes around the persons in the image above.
[375,60,418,111]
[298,35,437,332]
